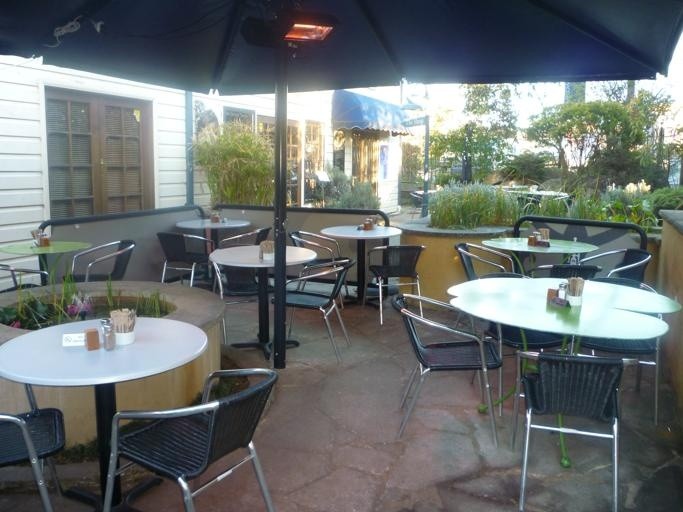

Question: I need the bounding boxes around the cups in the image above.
[567,294,582,308]
[115,330,135,346]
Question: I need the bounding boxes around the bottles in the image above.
[532,231,541,241]
[557,282,571,300]
[98,318,114,350]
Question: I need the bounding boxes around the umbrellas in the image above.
[0,0,682,369]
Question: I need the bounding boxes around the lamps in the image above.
[278,8,343,45]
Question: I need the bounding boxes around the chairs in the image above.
[75,238,138,284]
[1,260,22,293]
[391,293,502,449]
[158,221,425,361]
[0,384,69,511]
[455,237,660,421]
[105,367,279,511]
[510,347,640,509]
